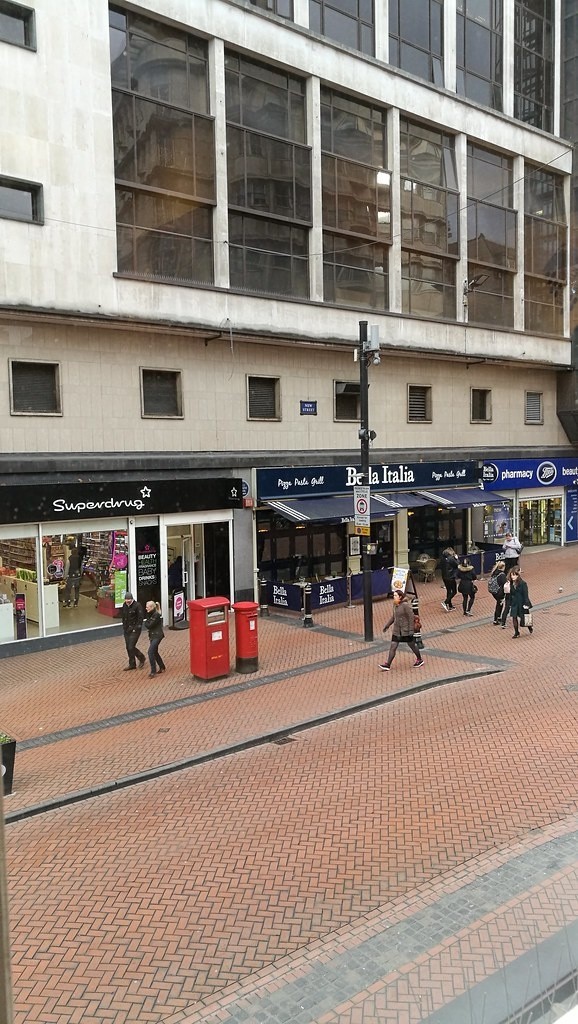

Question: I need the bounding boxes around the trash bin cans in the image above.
[188,596,229,681]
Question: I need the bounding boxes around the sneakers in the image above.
[379,662,391,670]
[413,658,424,667]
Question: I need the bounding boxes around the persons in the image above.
[169,556,187,598]
[502,533,520,574]
[67,536,90,565]
[144,601,166,678]
[457,559,477,616]
[491,561,507,625]
[509,571,533,638]
[500,566,521,628]
[122,592,146,671]
[441,548,459,611]
[63,549,81,607]
[379,590,424,670]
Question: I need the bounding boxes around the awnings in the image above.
[265,493,434,521]
[416,488,511,508]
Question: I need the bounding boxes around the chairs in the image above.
[416,553,437,584]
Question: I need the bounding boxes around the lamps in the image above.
[468,273,489,293]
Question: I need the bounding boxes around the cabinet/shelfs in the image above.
[1,530,128,577]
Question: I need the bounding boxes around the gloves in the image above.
[408,631,414,637]
[383,625,388,633]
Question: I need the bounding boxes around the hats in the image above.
[125,592,133,599]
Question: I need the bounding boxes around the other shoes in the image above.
[501,622,509,629]
[497,617,501,621]
[441,602,449,612]
[493,621,500,625]
[448,606,456,611]
[74,603,78,608]
[513,632,520,638]
[123,665,136,671]
[528,626,533,633]
[463,611,474,617]
[63,603,72,607]
[139,656,146,668]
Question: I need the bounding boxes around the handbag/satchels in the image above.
[413,614,422,632]
[524,608,533,627]
[514,538,524,554]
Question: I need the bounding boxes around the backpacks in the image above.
[488,572,504,595]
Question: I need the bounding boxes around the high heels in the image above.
[157,665,165,674]
[148,671,156,678]
